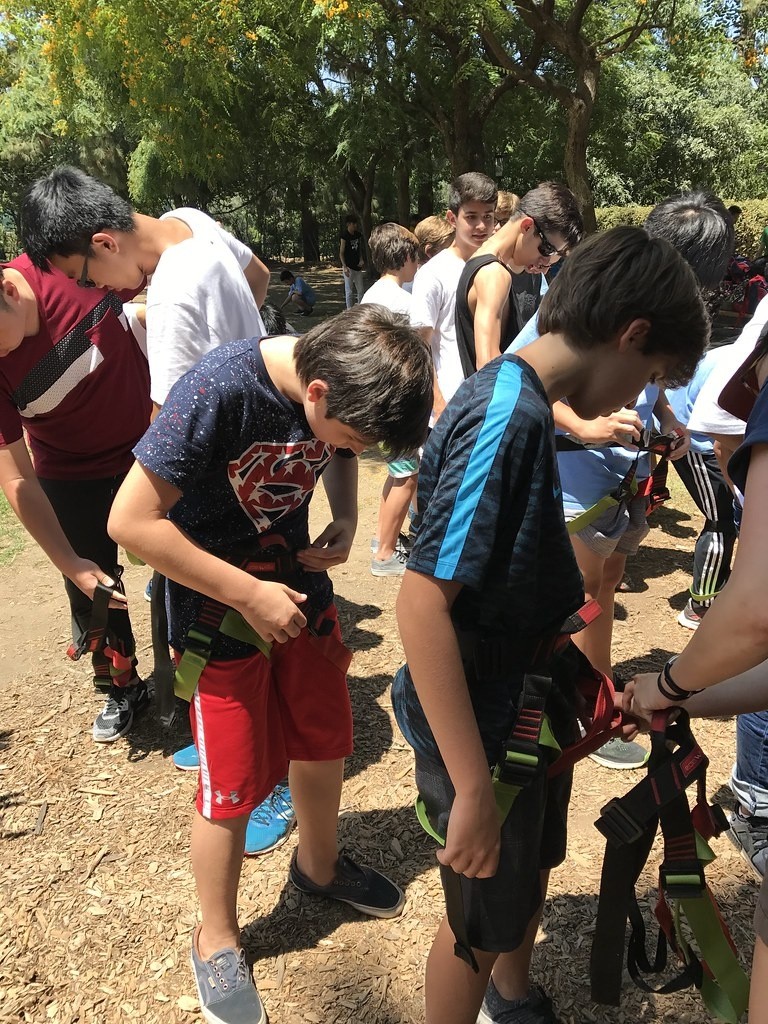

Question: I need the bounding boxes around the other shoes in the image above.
[300,307,314,317]
[677,598,708,630]
[615,571,635,591]
[291,309,305,315]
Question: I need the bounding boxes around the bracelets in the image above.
[657,673,690,701]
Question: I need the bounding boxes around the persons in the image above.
[361,172,768,1023]
[339,215,366,310]
[106,304,436,1024]
[124,269,316,358]
[14,165,270,855]
[0,253,154,741]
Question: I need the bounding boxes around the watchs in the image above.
[664,655,705,696]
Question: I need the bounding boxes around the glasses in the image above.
[76,224,100,288]
[493,218,508,227]
[520,207,559,257]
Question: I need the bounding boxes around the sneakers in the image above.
[725,801,768,880]
[244,778,297,856]
[370,533,415,553]
[172,744,200,771]
[92,675,148,742]
[370,550,408,576]
[289,853,406,919]
[480,975,563,1024]
[190,923,267,1024]
[577,718,651,770]
